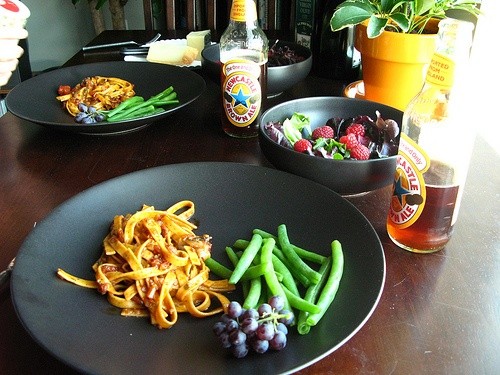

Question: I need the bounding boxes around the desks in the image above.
[0,30,499,375]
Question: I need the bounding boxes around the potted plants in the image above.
[329,0,482,111]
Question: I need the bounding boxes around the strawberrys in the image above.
[294,123,374,161]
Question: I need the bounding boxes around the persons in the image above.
[0,26,29,86]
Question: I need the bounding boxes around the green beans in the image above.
[205,223,343,335]
[99,85,179,121]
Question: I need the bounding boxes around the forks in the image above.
[82,33,161,50]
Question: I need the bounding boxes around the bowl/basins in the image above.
[259,97,403,197]
[202,40,312,99]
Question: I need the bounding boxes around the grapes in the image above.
[212,295,295,358]
[75,102,105,124]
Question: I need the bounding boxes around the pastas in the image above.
[57,199,235,328]
[55,75,136,116]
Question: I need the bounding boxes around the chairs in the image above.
[143,0,295,30]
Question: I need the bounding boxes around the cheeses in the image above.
[146,30,212,66]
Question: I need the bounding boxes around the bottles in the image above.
[386,18,476,255]
[220,0,269,139]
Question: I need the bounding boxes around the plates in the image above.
[8,160,390,375]
[3,61,212,136]
